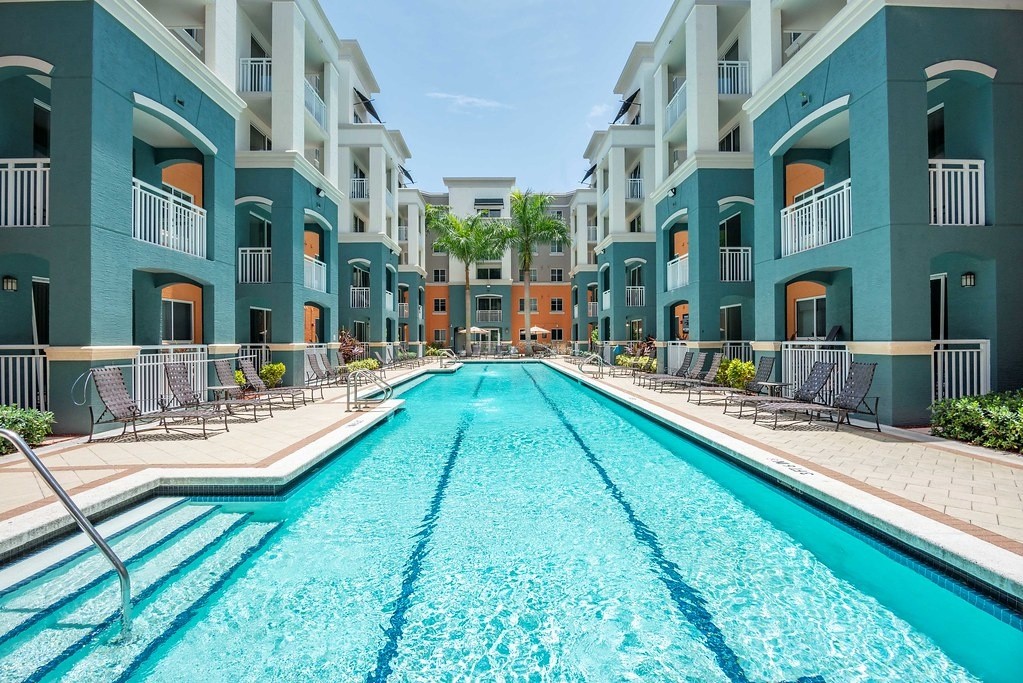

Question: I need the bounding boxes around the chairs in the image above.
[819,325,839,349]
[299,340,728,393]
[686,356,775,406]
[214,360,305,410]
[237,358,323,401]
[752,362,881,434]
[722,361,836,418]
[85,366,229,440]
[161,361,274,423]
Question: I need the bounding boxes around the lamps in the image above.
[505,328,508,333]
[316,187,325,196]
[961,272,976,287]
[2,277,17,291]
[486,284,490,291]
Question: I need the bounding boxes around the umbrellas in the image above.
[521,326,551,345]
[458,326,490,345]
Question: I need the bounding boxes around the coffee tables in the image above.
[206,385,241,416]
[757,380,792,395]
